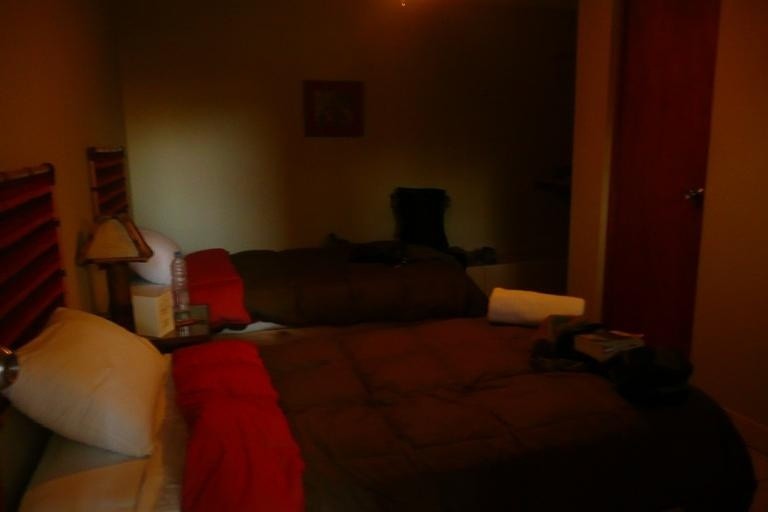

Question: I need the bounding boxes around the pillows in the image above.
[489,283,588,327]
[127,227,183,286]
[4,308,173,462]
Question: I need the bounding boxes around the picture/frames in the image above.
[302,79,367,137]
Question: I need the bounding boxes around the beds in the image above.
[2,313,760,510]
[120,238,485,331]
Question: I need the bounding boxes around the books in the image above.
[572,329,644,364]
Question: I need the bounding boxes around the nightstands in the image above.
[109,300,209,351]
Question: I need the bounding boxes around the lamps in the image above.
[78,214,154,310]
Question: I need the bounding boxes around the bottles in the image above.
[169,251,188,305]
